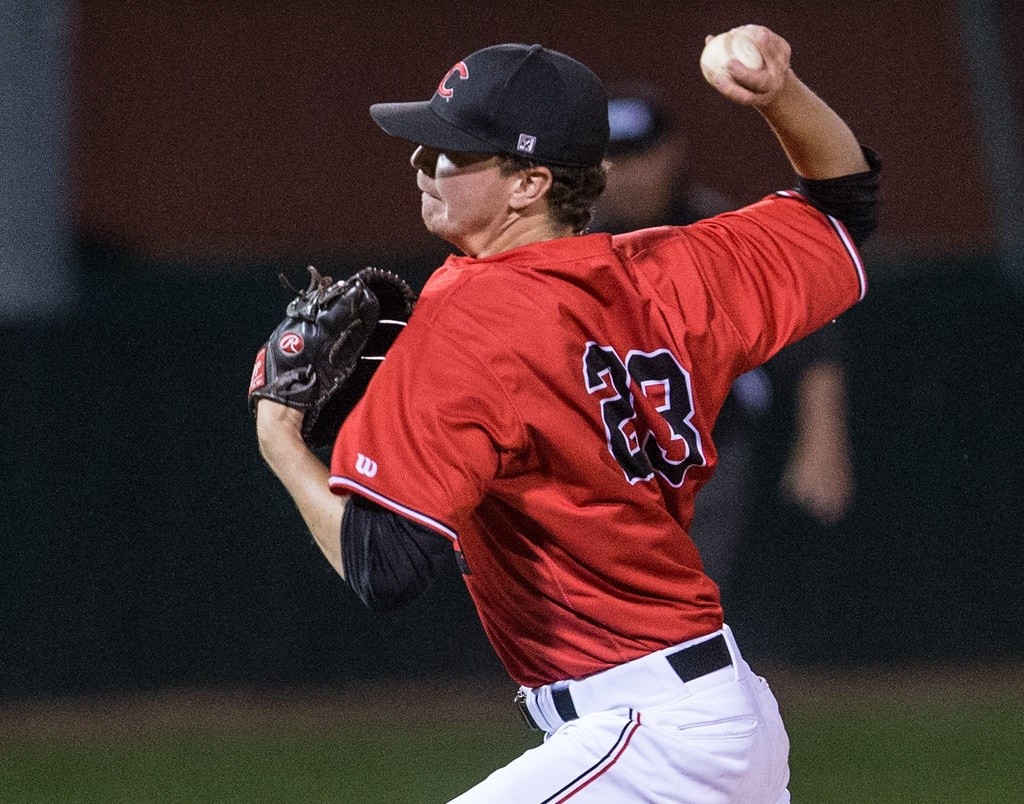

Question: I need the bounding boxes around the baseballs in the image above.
[700,31,764,86]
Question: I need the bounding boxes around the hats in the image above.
[370,44,612,167]
[606,100,671,155]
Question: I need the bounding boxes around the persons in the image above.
[595,98,852,593]
[248,24,884,804]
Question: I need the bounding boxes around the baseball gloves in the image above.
[245,263,418,447]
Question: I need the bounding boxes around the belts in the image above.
[516,631,733,731]
